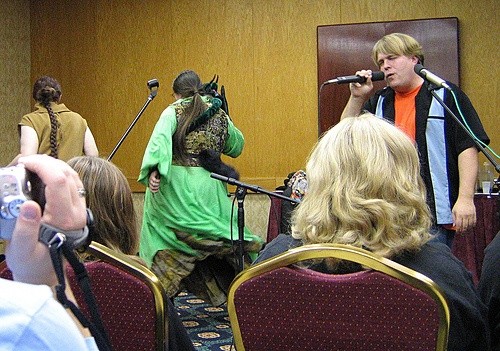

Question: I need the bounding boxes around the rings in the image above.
[77,188,87,197]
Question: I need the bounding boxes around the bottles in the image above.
[481,162,493,193]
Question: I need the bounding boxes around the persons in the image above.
[136,69,263,309]
[247,110,489,351]
[66,156,148,267]
[339,33,488,249]
[0,152,101,351]
[18,75,100,161]
[477,229,500,351]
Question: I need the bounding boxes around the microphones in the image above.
[324,71,385,86]
[413,64,451,90]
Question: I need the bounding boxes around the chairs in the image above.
[0,241,170,351]
[227,242,452,351]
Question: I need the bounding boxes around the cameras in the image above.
[0,166,48,241]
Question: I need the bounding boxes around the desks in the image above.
[266,191,500,287]
